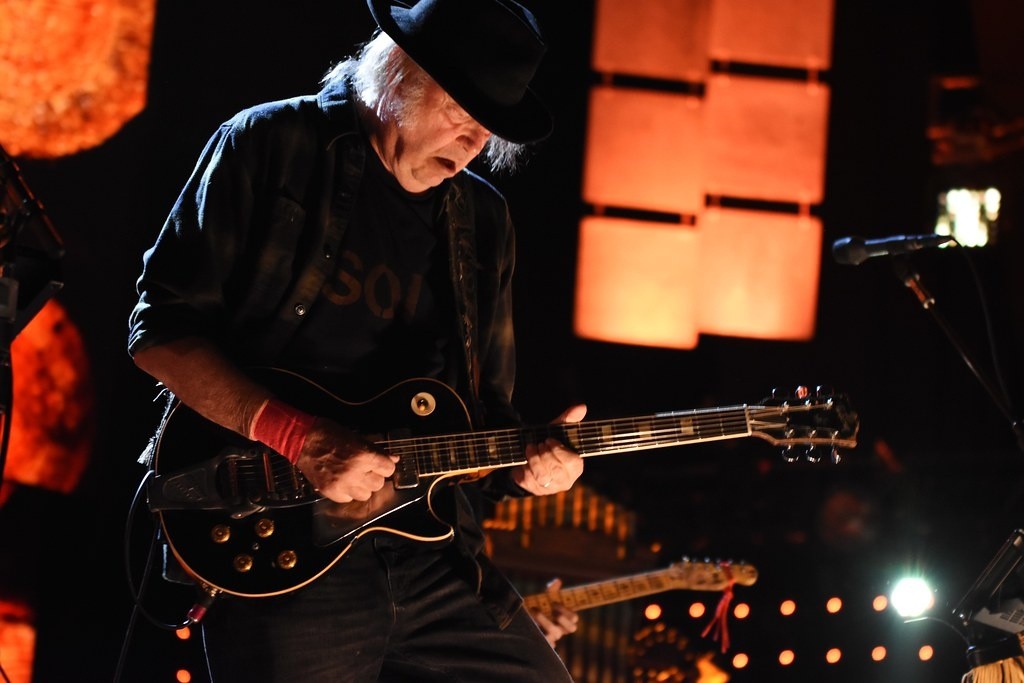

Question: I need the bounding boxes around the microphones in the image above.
[832,234,954,266]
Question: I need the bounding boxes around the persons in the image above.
[125,0,586,683]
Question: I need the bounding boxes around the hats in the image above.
[367,0,554,143]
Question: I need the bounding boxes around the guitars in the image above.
[154,360,863,598]
[522,555,758,628]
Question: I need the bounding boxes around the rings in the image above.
[541,480,551,487]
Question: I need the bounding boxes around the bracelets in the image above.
[246,395,313,466]
